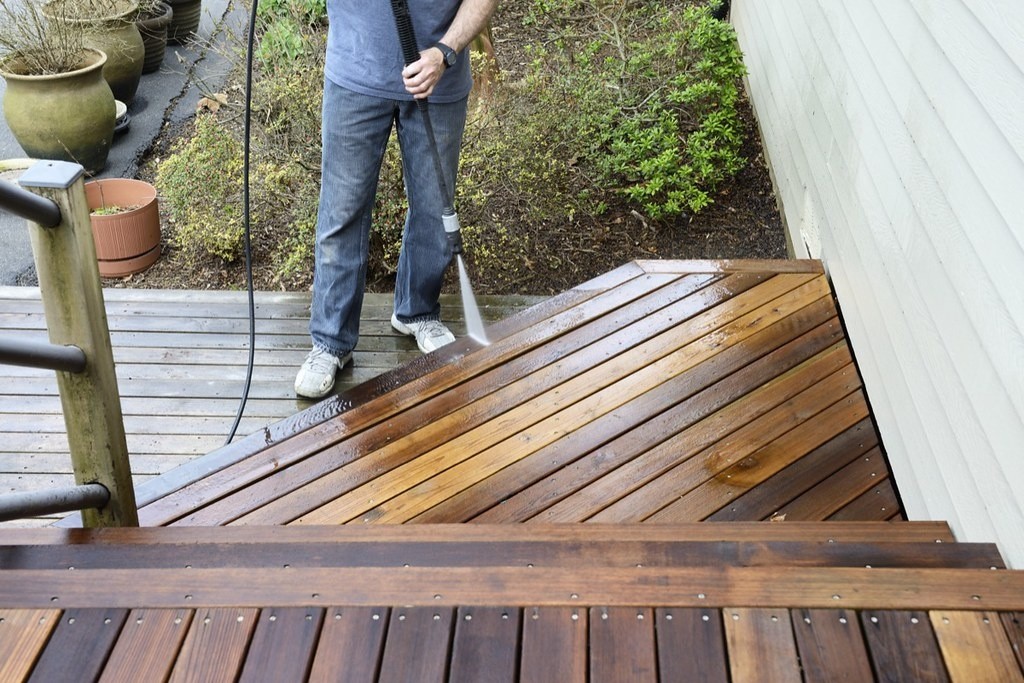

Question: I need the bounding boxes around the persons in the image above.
[294,0,499,397]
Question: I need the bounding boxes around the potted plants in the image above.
[0,0,173,179]
[57,139,161,277]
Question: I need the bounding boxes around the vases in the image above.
[162,0,201,47]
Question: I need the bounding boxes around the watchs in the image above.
[432,42,457,69]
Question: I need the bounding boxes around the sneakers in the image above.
[295,344,353,397]
[391,313,456,354]
